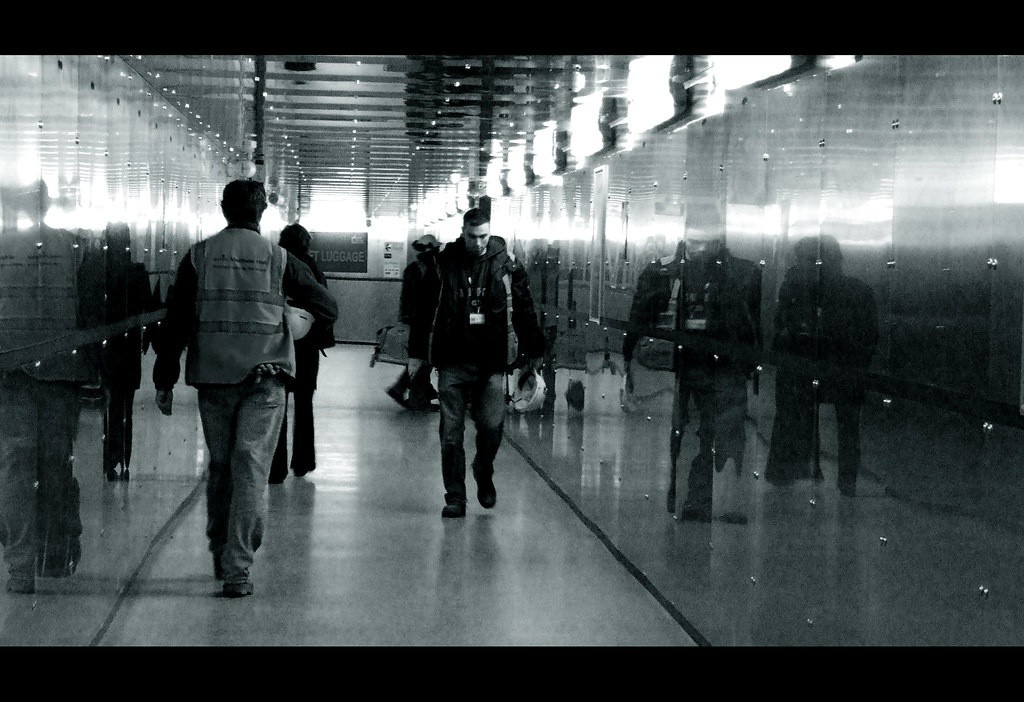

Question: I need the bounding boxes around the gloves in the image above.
[255,363,280,384]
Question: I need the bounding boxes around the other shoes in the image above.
[6,568,35,594]
[293,468,309,476]
[385,384,407,406]
[38,542,81,577]
[441,501,466,518]
[473,467,497,508]
[223,579,254,596]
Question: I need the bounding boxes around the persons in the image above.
[386,208,545,517]
[766,235,879,497]
[153,179,339,597]
[624,207,763,523]
[0,177,153,593]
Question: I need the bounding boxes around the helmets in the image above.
[283,300,313,340]
[417,234,443,253]
[514,367,546,411]
